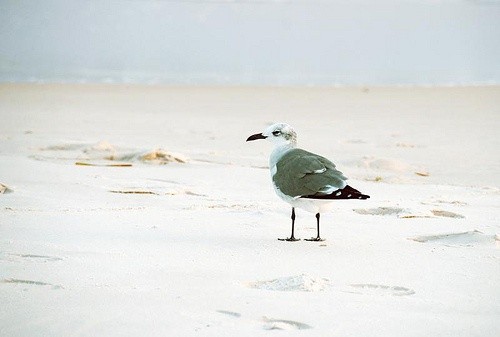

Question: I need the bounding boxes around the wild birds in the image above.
[246,123,370,241]
